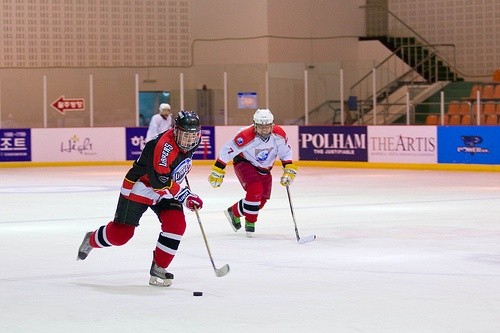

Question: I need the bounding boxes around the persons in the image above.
[76,110,204,287]
[144,103,173,148]
[207,109,297,233]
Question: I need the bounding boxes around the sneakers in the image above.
[245,218,255,237]
[77,229,97,260]
[225,206,242,232]
[149,251,174,287]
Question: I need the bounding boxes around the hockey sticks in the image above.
[184,174,230,277]
[286,182,316,244]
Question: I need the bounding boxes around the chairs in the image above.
[425,68,500,126]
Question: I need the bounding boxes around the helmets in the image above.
[254,109,274,138]
[175,110,201,152]
[159,103,171,111]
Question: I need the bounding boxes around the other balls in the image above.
[194,292,202,296]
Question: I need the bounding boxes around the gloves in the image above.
[209,164,225,188]
[280,165,298,186]
[183,192,203,211]
